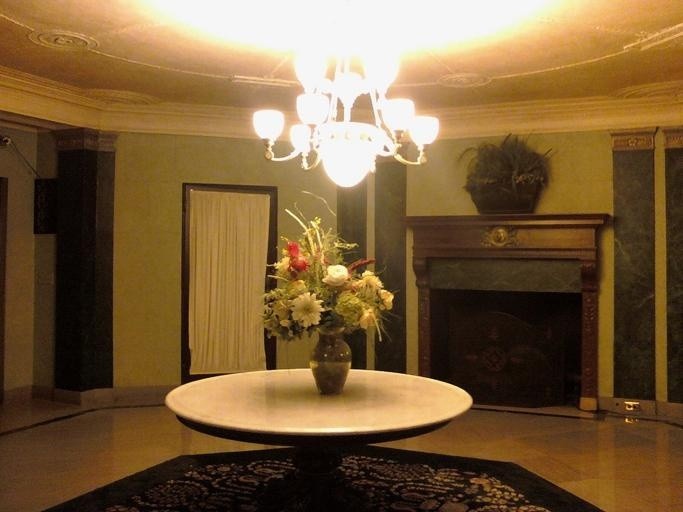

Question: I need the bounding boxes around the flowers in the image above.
[259,191,403,342]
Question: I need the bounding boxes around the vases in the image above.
[309,326,351,394]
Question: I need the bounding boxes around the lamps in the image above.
[252,0,439,187]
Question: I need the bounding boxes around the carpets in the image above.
[43,447,602,512]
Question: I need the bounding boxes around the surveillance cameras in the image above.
[2,135,12,147]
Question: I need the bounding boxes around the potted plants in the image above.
[457,128,555,215]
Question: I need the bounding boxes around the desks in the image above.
[164,368,474,512]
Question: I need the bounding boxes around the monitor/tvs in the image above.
[34,177,82,232]
